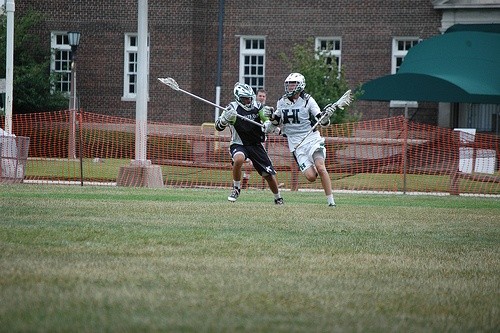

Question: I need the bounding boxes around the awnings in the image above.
[352,31,500,103]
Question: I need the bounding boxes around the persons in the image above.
[241,90,272,191]
[215,82,284,204]
[263,73,336,207]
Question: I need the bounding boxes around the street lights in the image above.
[67,31,81,159]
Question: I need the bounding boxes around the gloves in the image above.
[321,105,336,117]
[262,120,275,133]
[222,105,237,124]
[263,106,275,120]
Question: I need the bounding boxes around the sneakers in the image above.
[228,185,241,201]
[328,203,336,207]
[241,177,249,189]
[275,197,284,205]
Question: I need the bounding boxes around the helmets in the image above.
[284,72,306,96]
[234,81,256,111]
